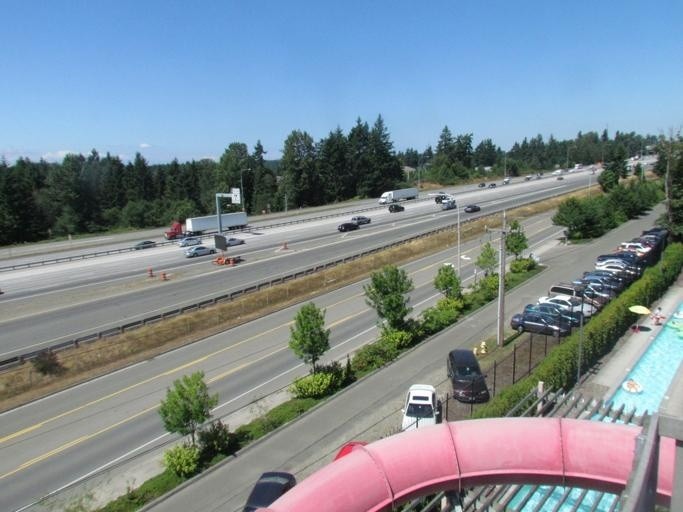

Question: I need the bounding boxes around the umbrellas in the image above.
[628,304,651,328]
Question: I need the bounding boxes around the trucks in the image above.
[164,211,248,240]
[378,188,418,205]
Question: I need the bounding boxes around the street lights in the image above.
[239,168,252,209]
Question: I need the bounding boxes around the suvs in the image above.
[389,204,404,212]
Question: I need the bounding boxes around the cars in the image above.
[435,191,456,210]
[338,216,371,232]
[511,226,669,338]
[446,349,489,403]
[241,471,297,512]
[334,441,369,461]
[179,237,216,257]
[226,237,245,246]
[477,163,583,188]
[465,205,480,212]
[134,240,157,250]
[401,384,440,431]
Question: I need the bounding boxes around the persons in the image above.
[653,307,661,325]
